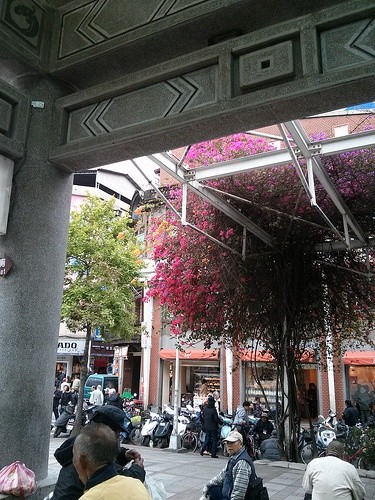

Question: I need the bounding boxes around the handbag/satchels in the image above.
[246,473,269,500]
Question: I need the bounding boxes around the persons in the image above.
[47,370,140,421]
[308,382,318,418]
[198,431,255,500]
[232,400,251,426]
[50,405,146,500]
[253,413,274,447]
[252,394,263,418]
[342,400,360,427]
[294,381,306,421]
[198,378,224,458]
[301,439,367,500]
[73,421,153,500]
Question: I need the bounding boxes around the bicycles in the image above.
[299,424,367,469]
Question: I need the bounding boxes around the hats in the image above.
[91,404,131,434]
[222,431,243,443]
[207,397,216,403]
[261,413,269,418]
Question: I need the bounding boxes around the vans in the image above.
[83,374,118,405]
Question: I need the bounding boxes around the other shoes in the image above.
[199,449,218,459]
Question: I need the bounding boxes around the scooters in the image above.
[317,415,339,448]
[53,406,87,438]
[117,393,264,461]
